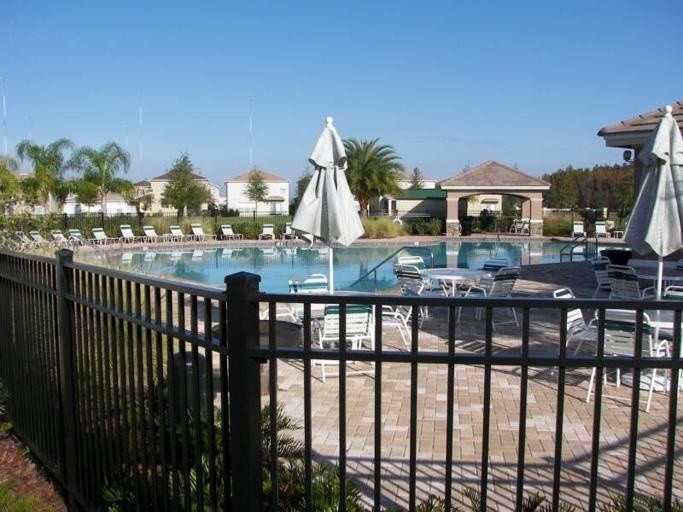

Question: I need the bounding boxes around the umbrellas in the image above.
[619,104,683,345]
[289,116,366,347]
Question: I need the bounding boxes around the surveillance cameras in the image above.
[624,151,632,160]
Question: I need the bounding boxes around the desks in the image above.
[211,318,300,395]
[420,267,492,296]
[592,309,675,330]
[634,268,682,282]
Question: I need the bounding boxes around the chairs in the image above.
[393,263,445,318]
[591,257,619,300]
[12,221,294,248]
[605,264,656,303]
[584,309,670,412]
[551,287,611,376]
[662,282,682,298]
[380,281,425,353]
[310,303,376,383]
[398,256,427,272]
[260,307,297,324]
[473,257,512,319]
[456,266,521,332]
[286,272,328,314]
[571,220,612,239]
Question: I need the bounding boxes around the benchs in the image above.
[508,218,530,235]
